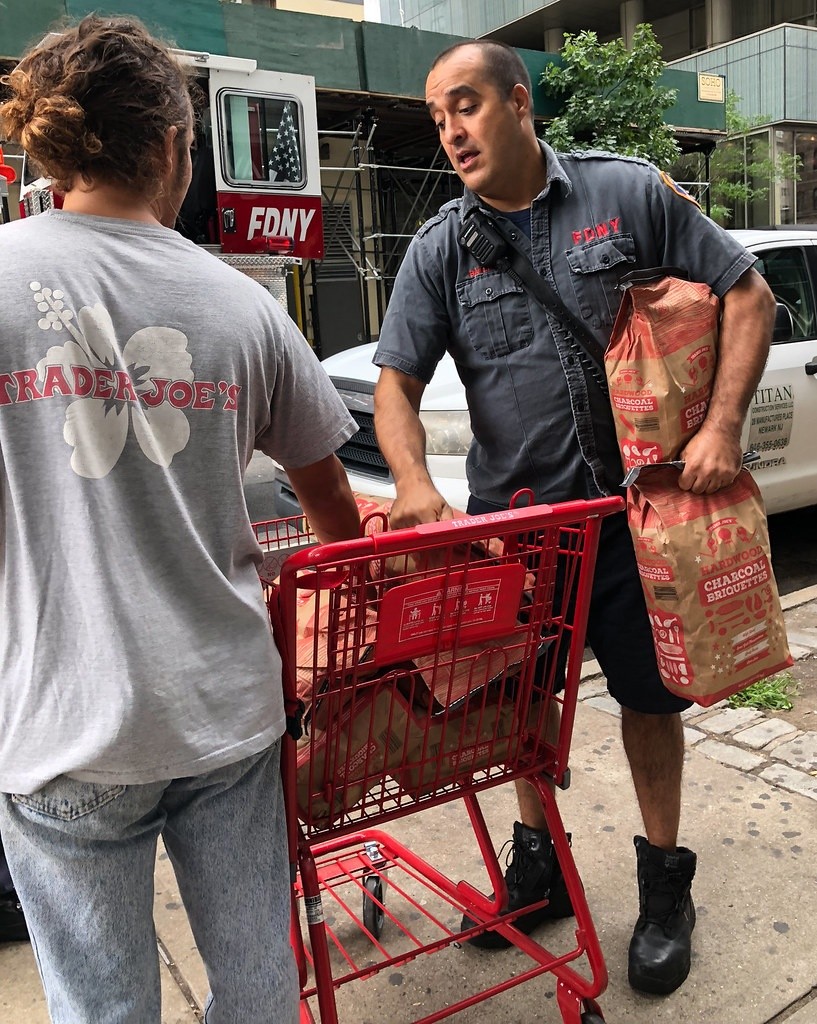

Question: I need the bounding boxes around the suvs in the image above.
[271,229,817,534]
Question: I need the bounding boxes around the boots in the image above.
[625,835,697,997]
[461,821,585,951]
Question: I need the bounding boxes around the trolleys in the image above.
[247,487,624,1023]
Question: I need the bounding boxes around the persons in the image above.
[367,41,784,1004]
[0,9,376,1024]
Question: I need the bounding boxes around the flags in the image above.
[267,101,300,183]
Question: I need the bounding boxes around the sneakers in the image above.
[0,891,31,941]
